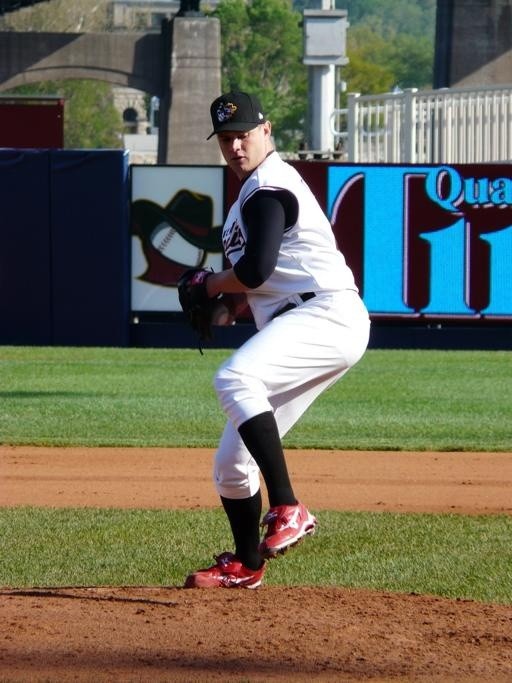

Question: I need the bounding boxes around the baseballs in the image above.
[210,304,229,327]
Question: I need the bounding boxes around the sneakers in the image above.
[184,551,265,591]
[258,503,317,561]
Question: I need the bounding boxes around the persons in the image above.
[177,92,370,588]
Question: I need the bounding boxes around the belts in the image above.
[267,293,315,320]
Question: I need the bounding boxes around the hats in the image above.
[207,91,266,142]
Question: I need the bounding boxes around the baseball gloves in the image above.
[178,269,234,353]
